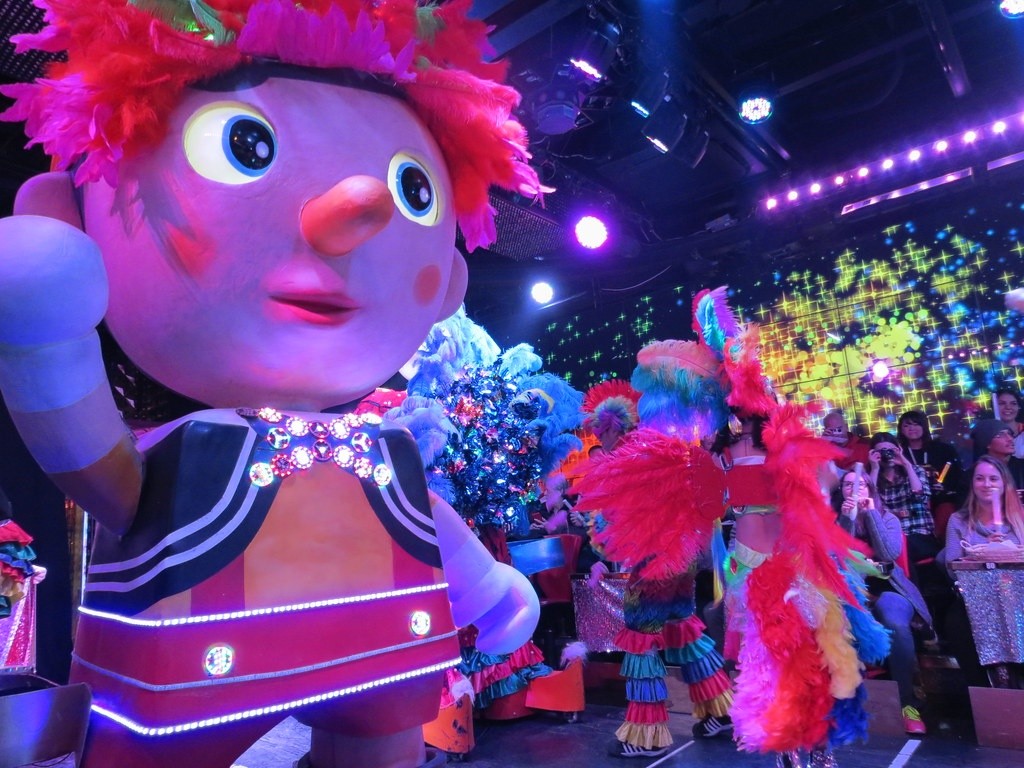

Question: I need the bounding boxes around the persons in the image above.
[707,389,838,768]
[863,432,933,596]
[834,467,936,734]
[990,389,1024,460]
[969,420,1024,508]
[817,410,874,476]
[534,441,610,586]
[568,422,734,761]
[944,452,1024,690]
[896,408,965,538]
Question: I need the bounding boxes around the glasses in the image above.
[841,482,872,488]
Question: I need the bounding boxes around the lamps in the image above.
[570,11,776,169]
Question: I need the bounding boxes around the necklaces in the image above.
[735,431,752,440]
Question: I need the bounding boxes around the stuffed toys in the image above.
[1,0,541,768]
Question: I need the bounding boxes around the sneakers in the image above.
[599,608,987,768]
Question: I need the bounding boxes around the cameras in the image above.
[872,447,896,461]
[832,426,844,434]
